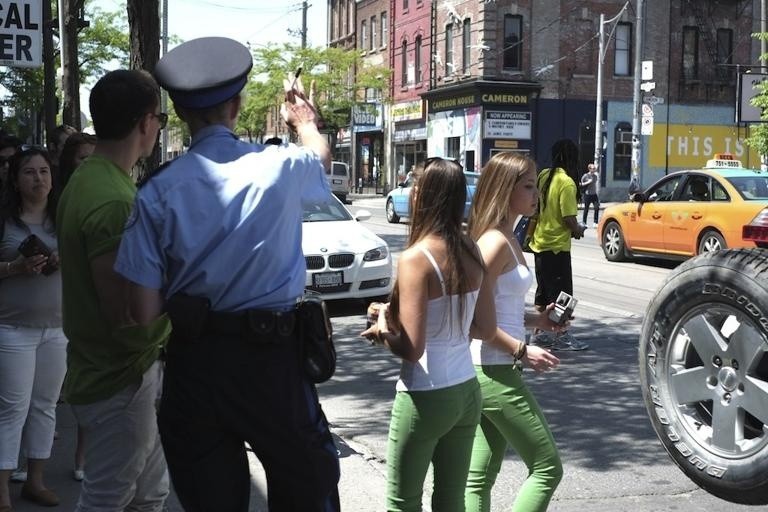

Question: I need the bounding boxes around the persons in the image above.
[580,163,600,228]
[49,67,174,510]
[264,137,283,147]
[406,164,417,179]
[1,118,100,512]
[528,136,591,351]
[465,153,573,512]
[111,35,343,510]
[360,158,496,512]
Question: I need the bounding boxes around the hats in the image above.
[154,37,252,108]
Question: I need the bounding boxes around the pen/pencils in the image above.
[369,336,375,345]
[290,68,302,89]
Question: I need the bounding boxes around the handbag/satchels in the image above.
[582,182,589,193]
[514,215,537,253]
[294,289,337,382]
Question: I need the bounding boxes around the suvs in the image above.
[638,241,768,506]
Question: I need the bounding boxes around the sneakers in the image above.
[551,331,589,351]
[530,332,555,347]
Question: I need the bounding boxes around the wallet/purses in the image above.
[18,234,58,275]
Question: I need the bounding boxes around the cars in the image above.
[386,164,481,220]
[298,192,394,308]
[598,153,767,261]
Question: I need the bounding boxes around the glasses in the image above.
[424,157,463,172]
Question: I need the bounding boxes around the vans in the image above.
[327,161,350,203]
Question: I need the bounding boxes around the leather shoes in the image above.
[10,467,83,506]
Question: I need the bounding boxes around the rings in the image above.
[551,326,555,331]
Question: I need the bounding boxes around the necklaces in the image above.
[503,227,516,240]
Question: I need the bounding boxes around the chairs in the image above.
[691,181,707,199]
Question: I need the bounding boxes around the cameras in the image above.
[548,291,578,326]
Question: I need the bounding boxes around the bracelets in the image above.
[375,329,386,349]
[511,339,529,367]
